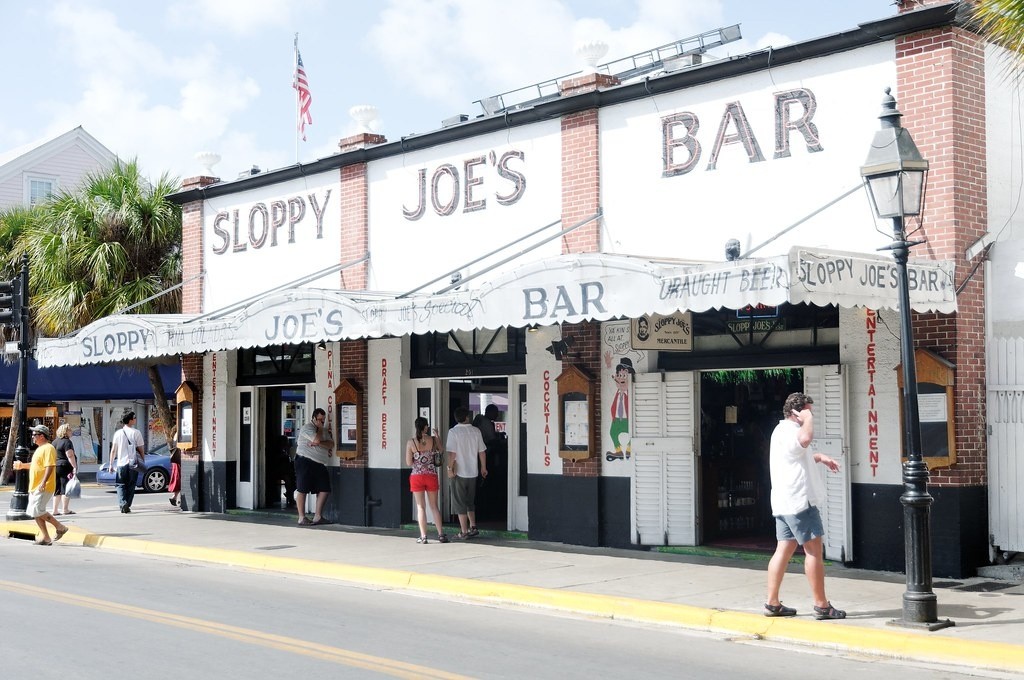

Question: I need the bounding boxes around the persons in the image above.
[13,425,68,546]
[763,392,846,620]
[52,423,77,515]
[271,436,296,504]
[473,402,507,504]
[167,425,181,506]
[286,405,299,436]
[446,408,487,540]
[406,417,449,544]
[109,411,145,513]
[294,408,334,526]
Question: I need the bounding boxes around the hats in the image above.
[30,425,50,436]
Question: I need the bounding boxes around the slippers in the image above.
[298,517,316,526]
[53,513,63,515]
[55,527,68,541]
[64,511,77,515]
[310,516,333,525]
[33,539,53,546]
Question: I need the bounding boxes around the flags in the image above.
[292,47,312,142]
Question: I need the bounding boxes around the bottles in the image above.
[34,488,40,496]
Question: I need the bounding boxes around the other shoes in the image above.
[169,497,177,506]
[120,502,130,513]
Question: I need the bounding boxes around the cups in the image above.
[718,500,728,508]
[737,497,755,506]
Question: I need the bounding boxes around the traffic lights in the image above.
[0,276,20,323]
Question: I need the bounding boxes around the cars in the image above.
[97,441,172,493]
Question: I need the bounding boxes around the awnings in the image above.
[36,177,959,369]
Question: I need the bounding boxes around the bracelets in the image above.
[448,468,453,470]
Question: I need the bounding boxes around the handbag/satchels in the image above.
[65,474,81,499]
[128,443,147,473]
[432,436,445,466]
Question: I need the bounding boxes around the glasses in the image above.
[31,434,41,436]
[133,417,136,419]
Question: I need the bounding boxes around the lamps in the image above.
[547,335,582,362]
[318,341,327,351]
[528,324,537,331]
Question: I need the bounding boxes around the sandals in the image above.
[763,601,797,617]
[467,526,479,536]
[451,531,470,540]
[438,534,448,543]
[813,601,846,619]
[416,535,427,544]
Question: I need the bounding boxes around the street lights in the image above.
[860,88,956,631]
